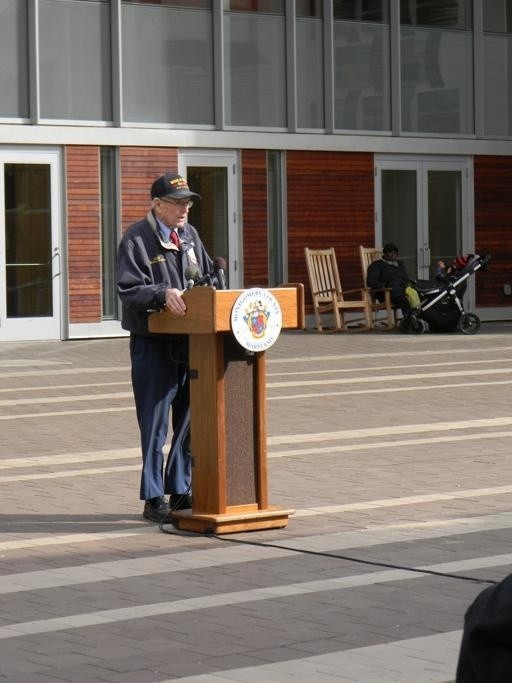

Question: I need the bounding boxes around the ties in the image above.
[169,233,181,244]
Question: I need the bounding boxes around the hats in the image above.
[151,172,201,200]
[457,256,467,267]
[383,243,399,253]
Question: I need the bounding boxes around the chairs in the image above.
[359,245,403,332]
[304,247,373,333]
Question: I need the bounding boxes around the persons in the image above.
[367,244,410,318]
[116,175,222,522]
[433,255,468,288]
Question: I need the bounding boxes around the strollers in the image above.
[398,251,493,335]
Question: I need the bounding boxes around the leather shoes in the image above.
[142,495,192,523]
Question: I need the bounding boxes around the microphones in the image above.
[212,256,227,290]
[184,267,199,291]
